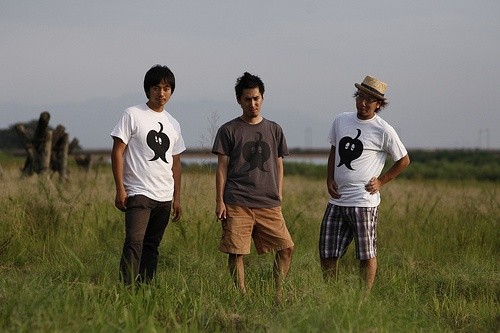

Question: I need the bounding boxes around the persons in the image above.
[319,75,411,299]
[110,64,186,290]
[211,71,295,301]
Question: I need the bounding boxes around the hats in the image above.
[354,74,389,100]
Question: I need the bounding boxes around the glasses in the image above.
[356,92,380,103]
